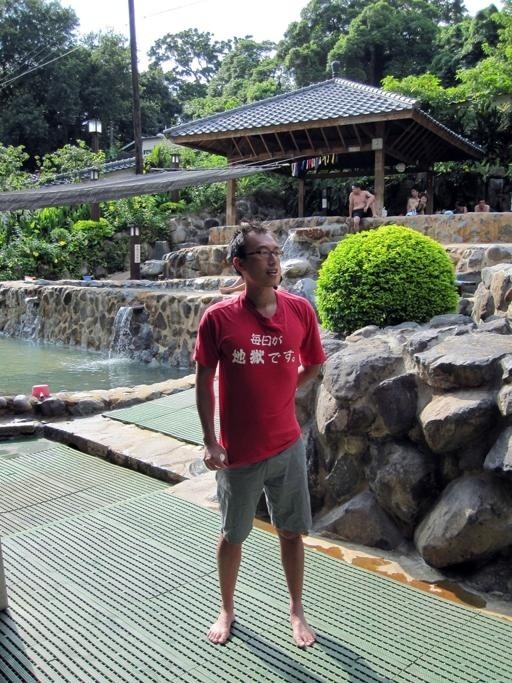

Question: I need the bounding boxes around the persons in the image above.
[405,187,421,217]
[452,201,467,214]
[473,197,490,212]
[347,182,379,234]
[399,191,428,216]
[194,223,328,649]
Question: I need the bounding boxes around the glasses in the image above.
[238,246,285,259]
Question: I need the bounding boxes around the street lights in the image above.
[86,111,103,154]
[169,150,181,202]
[89,166,101,220]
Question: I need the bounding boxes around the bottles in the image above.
[188,452,227,481]
